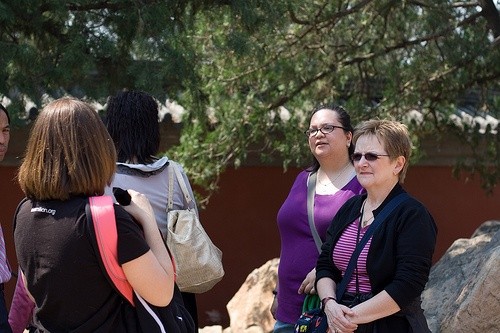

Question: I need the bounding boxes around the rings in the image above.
[335,330,338,333]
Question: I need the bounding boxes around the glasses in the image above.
[351,152,390,161]
[304,125,348,137]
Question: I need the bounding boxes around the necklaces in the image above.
[363,216,373,227]
[317,161,351,186]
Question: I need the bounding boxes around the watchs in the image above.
[319,297,336,315]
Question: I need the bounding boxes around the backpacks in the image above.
[12,194,196,333]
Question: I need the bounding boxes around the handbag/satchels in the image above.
[166,159,225,294]
[293,308,329,333]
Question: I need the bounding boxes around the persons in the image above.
[12,98,195,333]
[0,104,13,333]
[314,120,438,333]
[270,104,367,333]
[102,91,198,333]
